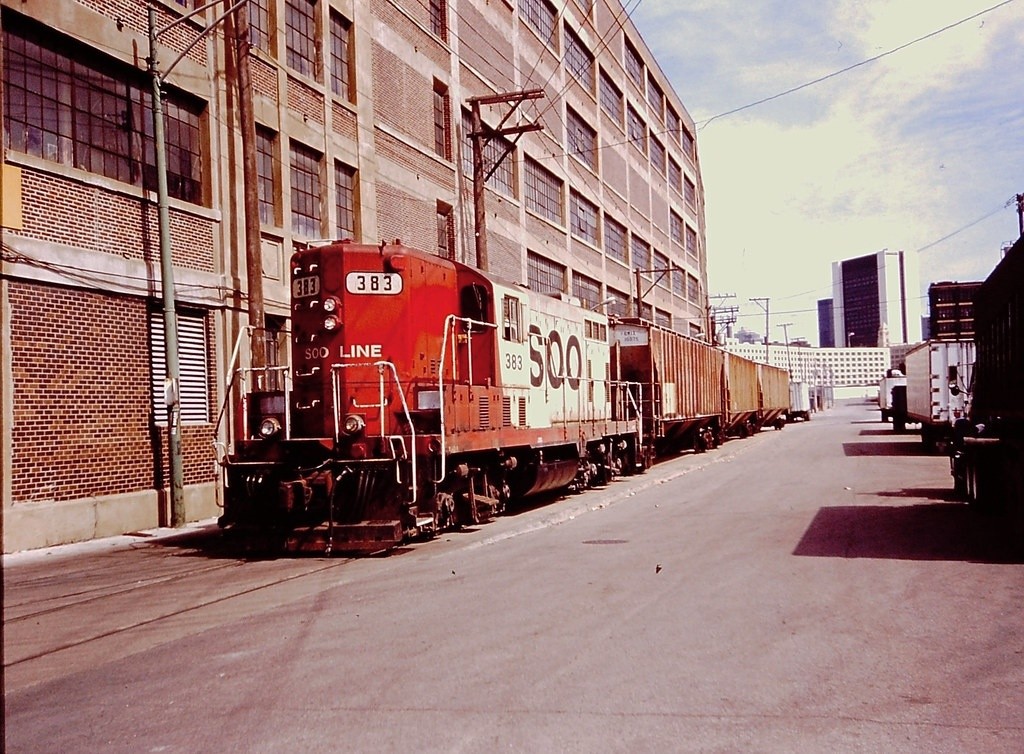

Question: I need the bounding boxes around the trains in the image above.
[215,235,815,559]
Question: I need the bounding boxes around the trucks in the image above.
[905,337,978,448]
[936,229,1024,517]
[877,375,909,422]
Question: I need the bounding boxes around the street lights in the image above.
[847,332,855,348]
